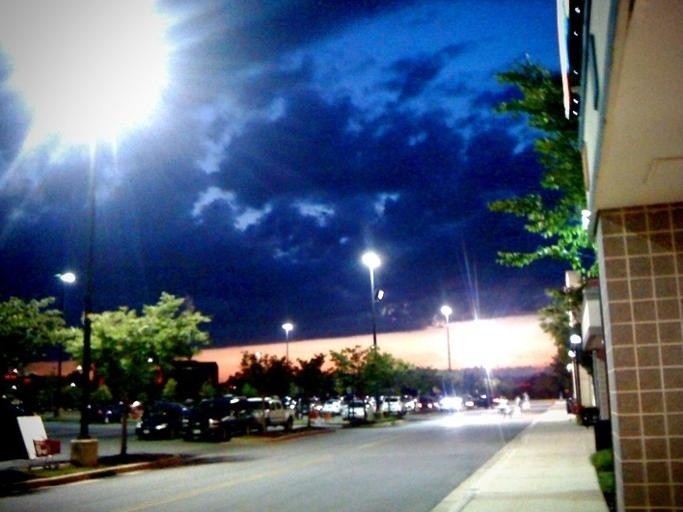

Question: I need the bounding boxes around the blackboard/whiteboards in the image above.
[17,415,55,470]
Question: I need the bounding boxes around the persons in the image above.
[495,390,529,417]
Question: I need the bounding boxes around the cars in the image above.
[88,401,128,423]
[311,395,420,425]
[135,394,253,444]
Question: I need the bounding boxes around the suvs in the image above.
[248,397,296,432]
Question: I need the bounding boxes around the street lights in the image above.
[362,251,384,350]
[69,29,104,437]
[441,306,453,371]
[283,323,293,363]
[56,271,75,419]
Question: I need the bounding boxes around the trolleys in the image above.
[24,440,60,470]
